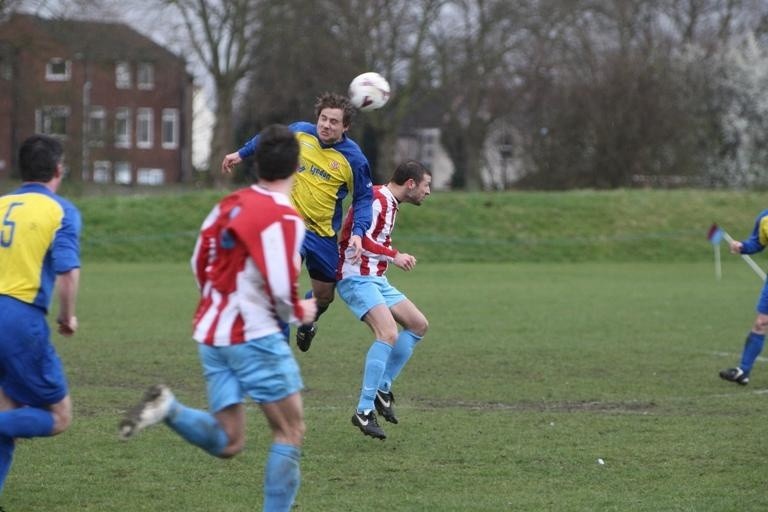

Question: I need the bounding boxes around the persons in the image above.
[333,160,432,441]
[120,123,316,511]
[719,209,768,384]
[221,92,373,352]
[0,135,83,512]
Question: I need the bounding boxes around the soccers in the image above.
[349,72,390,113]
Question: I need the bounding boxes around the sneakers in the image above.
[374,389,399,426]
[718,367,749,385]
[296,320,318,352]
[119,384,172,439]
[352,413,385,438]
